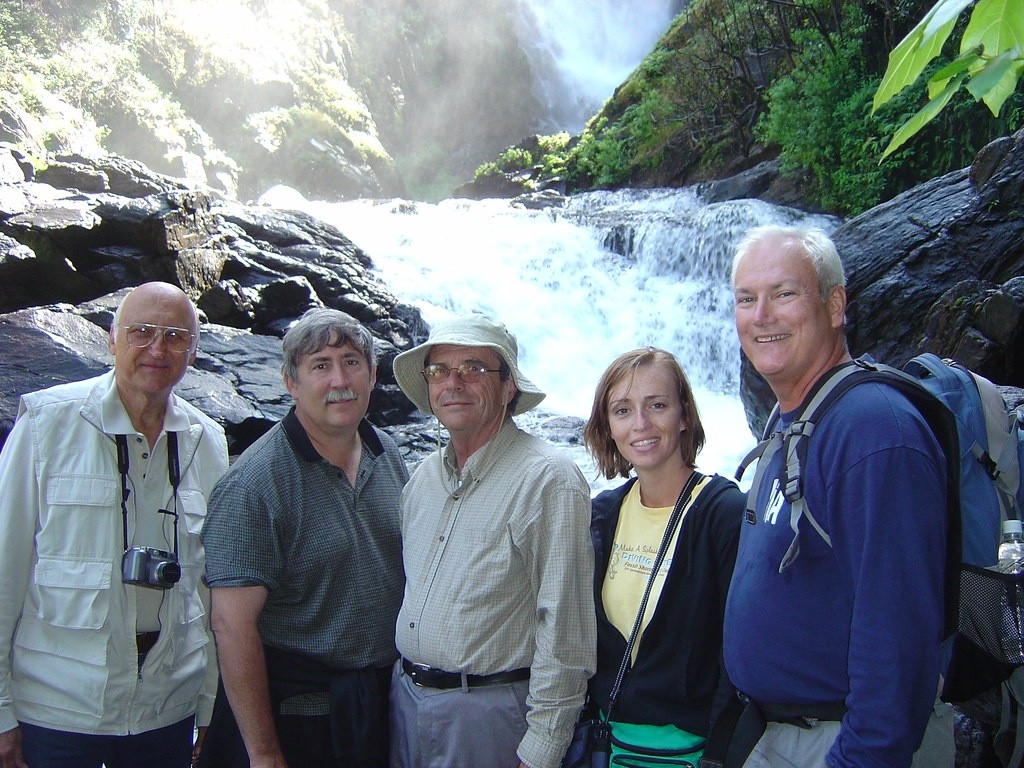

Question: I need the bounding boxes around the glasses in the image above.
[117,324,197,353]
[421,363,502,383]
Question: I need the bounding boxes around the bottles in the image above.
[996,520,1024,663]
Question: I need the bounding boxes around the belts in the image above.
[137,631,160,654]
[769,716,812,729]
[398,656,531,690]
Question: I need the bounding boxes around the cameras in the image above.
[122,546,181,590]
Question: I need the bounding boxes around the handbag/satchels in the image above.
[562,718,611,768]
[597,710,706,768]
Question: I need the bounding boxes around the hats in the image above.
[392,309,546,418]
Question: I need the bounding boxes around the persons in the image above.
[198,309,411,768]
[724,225,955,768]
[581,348,746,768]
[0,281,232,768]
[387,310,597,768]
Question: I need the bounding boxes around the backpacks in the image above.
[735,351,1024,702]
